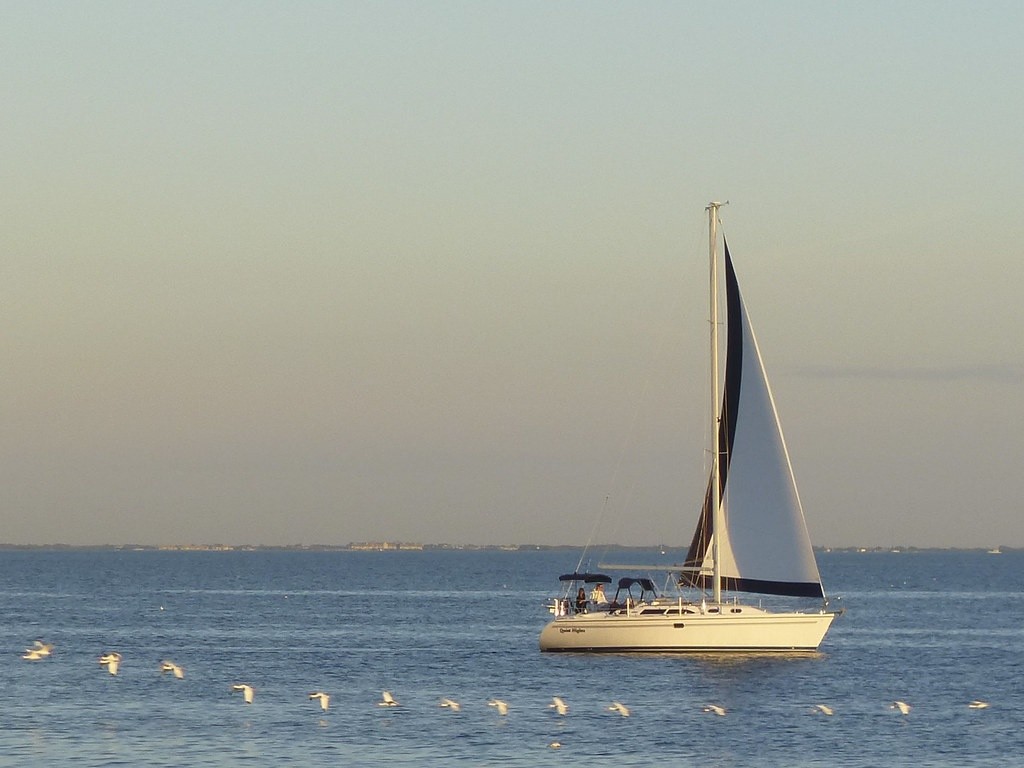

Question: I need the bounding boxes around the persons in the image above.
[590,583,609,606]
[575,587,587,614]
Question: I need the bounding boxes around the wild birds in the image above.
[812,704,833,716]
[159,662,184,679]
[488,699,508,715]
[608,703,630,716]
[550,743,560,747]
[703,704,726,716]
[26,641,52,655]
[21,653,41,660]
[890,701,911,715]
[233,685,254,704]
[309,693,329,712]
[969,700,989,708]
[441,699,460,712]
[98,652,122,675]
[549,697,569,715]
[378,692,400,707]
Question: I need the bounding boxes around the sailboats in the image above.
[539,200,845,655]
